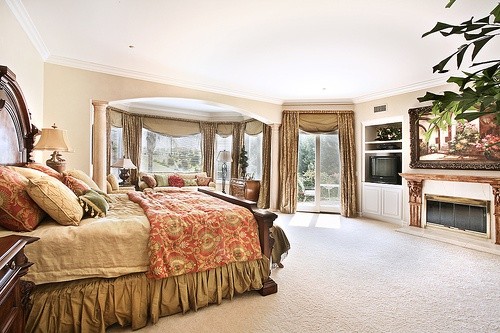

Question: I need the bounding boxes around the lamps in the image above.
[216,150,233,194]
[34,122,70,171]
[110,156,138,187]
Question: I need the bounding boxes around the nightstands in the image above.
[0,234,41,333]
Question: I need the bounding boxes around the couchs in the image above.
[107,179,135,194]
[135,171,216,192]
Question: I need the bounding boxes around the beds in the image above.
[0,65,290,333]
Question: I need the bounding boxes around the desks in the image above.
[320,183,340,201]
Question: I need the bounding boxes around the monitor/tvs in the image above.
[368,155,402,185]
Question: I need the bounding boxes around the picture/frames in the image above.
[408,105,500,170]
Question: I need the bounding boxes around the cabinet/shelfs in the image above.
[365,140,402,153]
[231,178,260,201]
[362,184,403,219]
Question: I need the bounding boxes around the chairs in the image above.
[298,179,315,205]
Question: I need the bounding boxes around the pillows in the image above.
[0,161,115,232]
[141,172,157,187]
[154,173,169,187]
[183,179,198,186]
[168,175,185,188]
[196,175,213,187]
[107,174,119,190]
